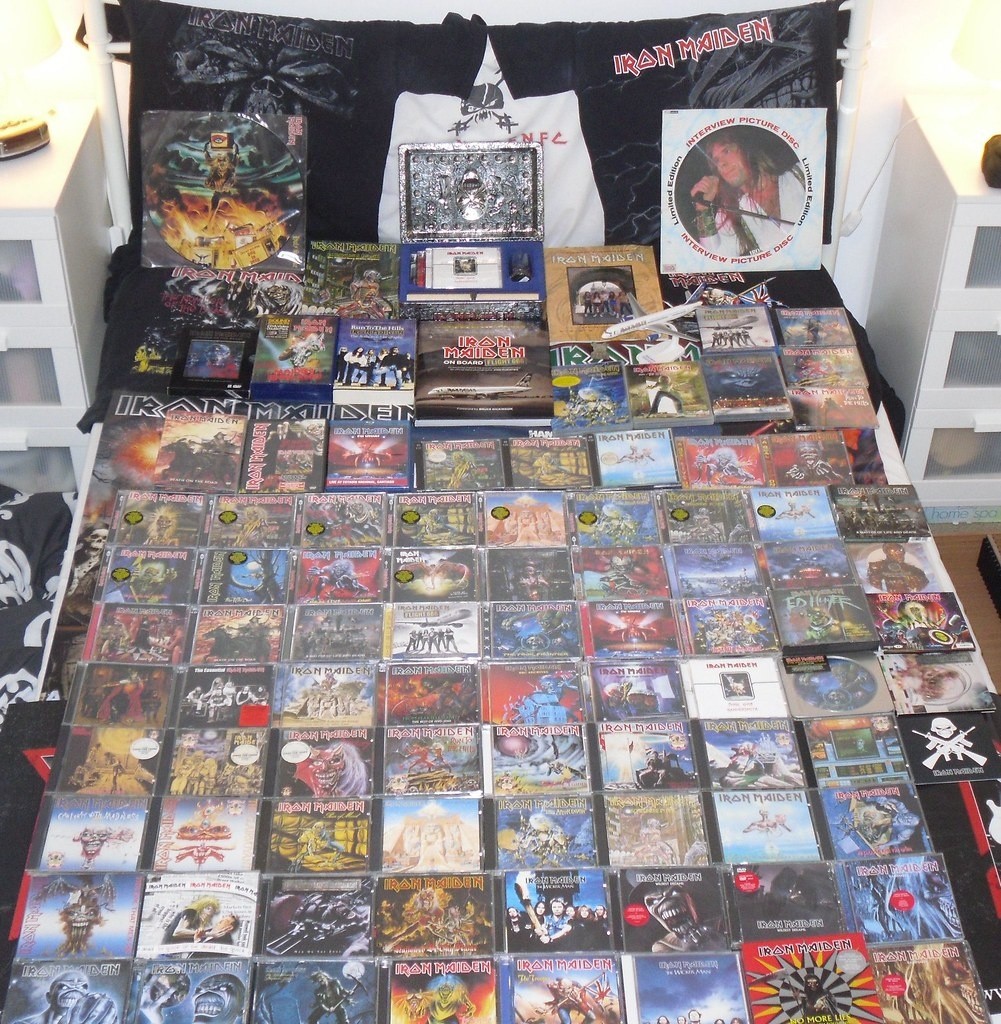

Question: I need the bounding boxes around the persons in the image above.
[690,127,806,257]
[334,346,414,390]
[583,290,634,318]
[506,898,612,952]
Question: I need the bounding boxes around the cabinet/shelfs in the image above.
[865,86,1001,535]
[0,106,114,503]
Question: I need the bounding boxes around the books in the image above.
[398,241,554,427]
[332,319,417,406]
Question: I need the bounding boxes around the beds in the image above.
[0,0,1001,1024]
